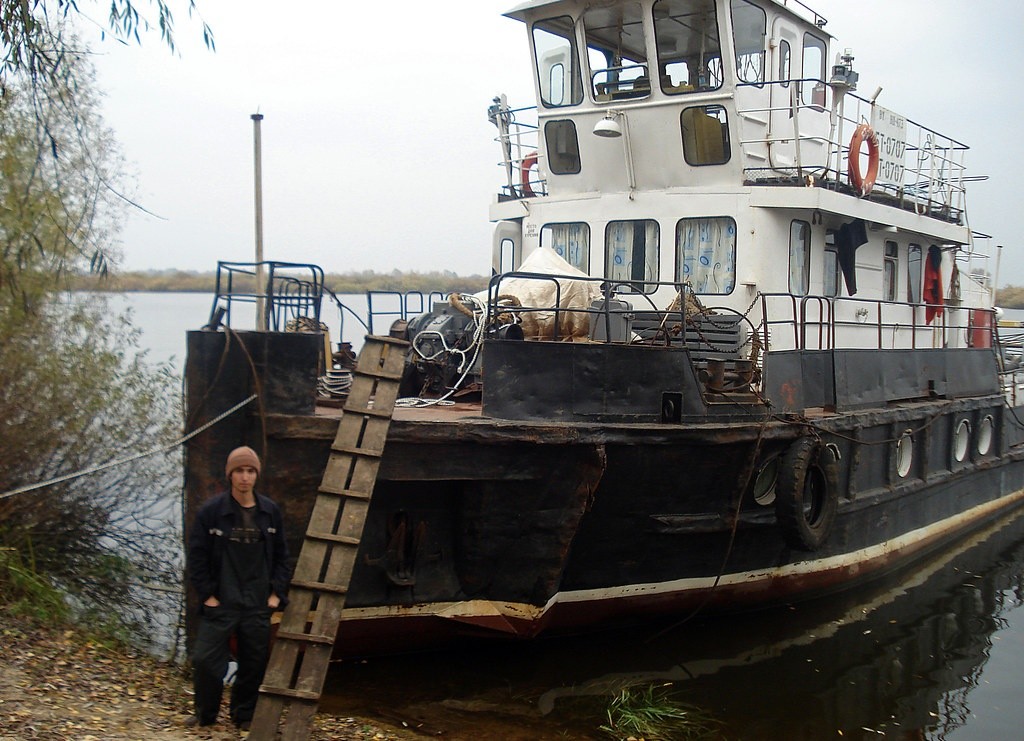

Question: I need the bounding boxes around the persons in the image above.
[186,446,291,736]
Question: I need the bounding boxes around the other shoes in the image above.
[183,716,201,727]
[241,721,251,730]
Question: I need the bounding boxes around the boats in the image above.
[179,0,1024,667]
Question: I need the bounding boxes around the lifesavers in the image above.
[519,152,539,198]
[775,434,842,553]
[848,124,879,195]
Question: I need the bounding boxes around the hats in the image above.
[225,446,260,483]
